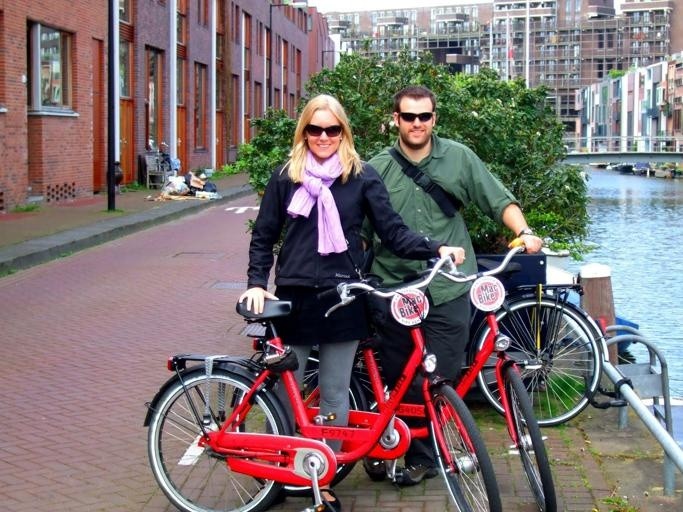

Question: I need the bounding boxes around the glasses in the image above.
[399,111,433,122]
[306,123,343,137]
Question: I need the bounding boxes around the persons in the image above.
[357,84,544,486]
[238,93,465,511]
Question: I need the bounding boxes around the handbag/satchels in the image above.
[362,272,392,340]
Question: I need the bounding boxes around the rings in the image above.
[462,257,466,261]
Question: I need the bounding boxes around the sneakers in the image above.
[396,464,439,487]
[363,456,385,481]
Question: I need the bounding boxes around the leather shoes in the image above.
[314,489,341,512]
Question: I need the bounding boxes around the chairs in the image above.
[145,156,177,191]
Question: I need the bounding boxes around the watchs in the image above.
[516,228,533,238]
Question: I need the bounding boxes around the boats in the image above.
[591,162,683,180]
